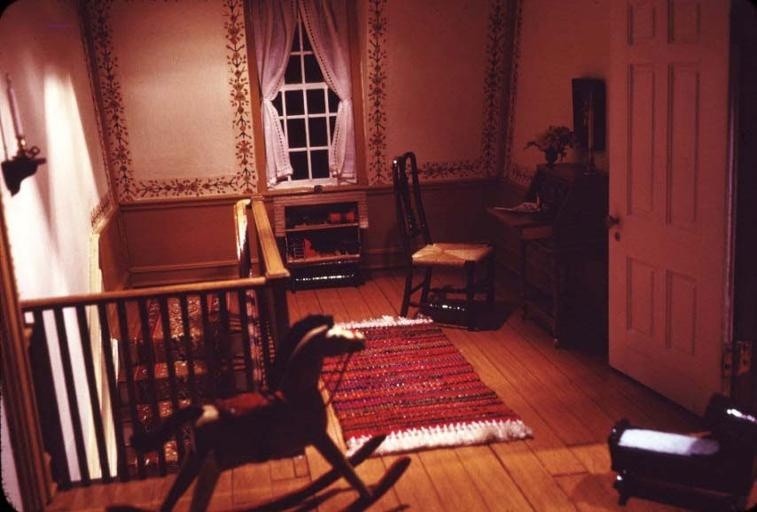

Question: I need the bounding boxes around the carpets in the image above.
[319,315,534,463]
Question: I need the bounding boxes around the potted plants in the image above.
[525,124,594,164]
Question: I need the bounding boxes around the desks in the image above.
[489,204,609,347]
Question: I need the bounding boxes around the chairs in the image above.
[393,151,499,331]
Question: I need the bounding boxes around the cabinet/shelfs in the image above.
[271,190,369,293]
